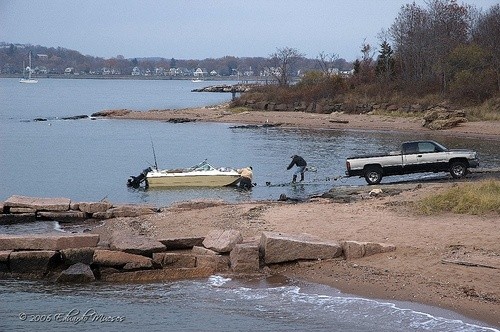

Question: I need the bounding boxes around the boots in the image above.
[300,173,304,181]
[292,175,297,184]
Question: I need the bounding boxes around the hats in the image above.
[290,153,297,157]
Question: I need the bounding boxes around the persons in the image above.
[286,154,306,182]
[238,167,253,190]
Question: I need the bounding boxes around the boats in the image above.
[127,158,241,188]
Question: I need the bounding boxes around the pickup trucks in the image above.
[342,139,480,184]
